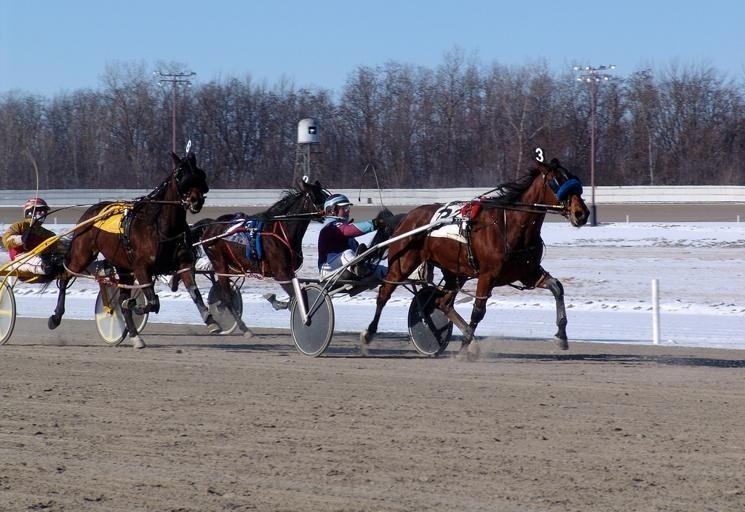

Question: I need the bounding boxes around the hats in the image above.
[335,201,353,207]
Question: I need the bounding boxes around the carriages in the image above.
[273,158,590,361]
[0,150,222,350]
[127,180,332,338]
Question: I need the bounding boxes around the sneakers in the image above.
[354,243,368,276]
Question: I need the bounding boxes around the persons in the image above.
[317,192,391,283]
[4,198,69,276]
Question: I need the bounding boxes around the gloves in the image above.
[372,208,394,230]
[22,231,30,242]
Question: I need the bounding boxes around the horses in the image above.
[168,177,334,340]
[358,157,591,362]
[38,149,223,352]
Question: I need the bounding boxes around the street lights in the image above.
[572,63,618,227]
[152,70,197,171]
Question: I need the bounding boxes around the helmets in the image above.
[324,194,352,214]
[24,198,50,225]
